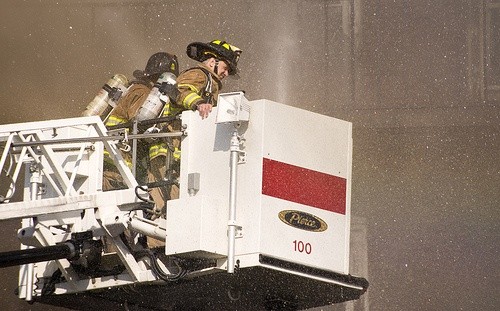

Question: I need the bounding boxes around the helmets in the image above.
[186,40,243,75]
[133,52,179,77]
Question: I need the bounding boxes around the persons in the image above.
[138,39,242,249]
[102,52,179,253]
